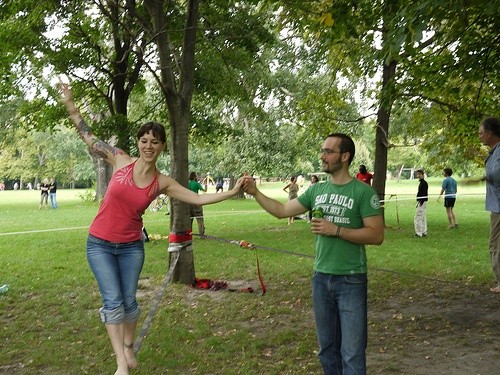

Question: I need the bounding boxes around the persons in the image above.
[39,178,49,209]
[284,176,299,225]
[307,175,318,223]
[414,170,428,237]
[251,175,255,181]
[437,169,457,228]
[62,89,251,375]
[221,180,223,185]
[37,183,40,190]
[28,182,32,190]
[187,172,207,236]
[0,182,5,190]
[48,178,57,208]
[14,183,18,190]
[216,183,223,193]
[241,133,384,375]
[478,118,500,292]
[355,165,374,185]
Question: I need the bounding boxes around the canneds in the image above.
[312,206,324,227]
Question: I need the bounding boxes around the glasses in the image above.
[320,148,342,155]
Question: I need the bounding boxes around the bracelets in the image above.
[69,110,78,117]
[336,226,341,238]
